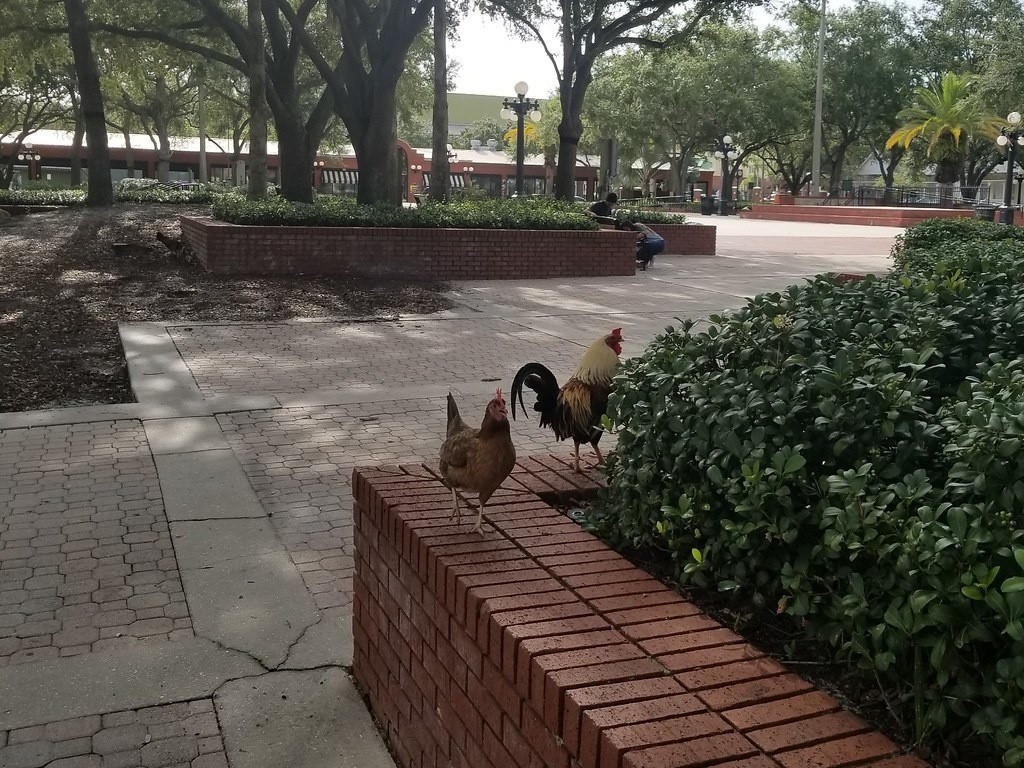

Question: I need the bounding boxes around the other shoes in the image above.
[650,255,655,265]
[640,261,648,271]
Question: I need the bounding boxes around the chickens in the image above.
[438,386,516,540]
[510,327,625,474]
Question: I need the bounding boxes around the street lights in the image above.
[996,111,1024,225]
[499,80,542,196]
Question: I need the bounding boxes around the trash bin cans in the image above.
[975,204,996,222]
[700,196,714,215]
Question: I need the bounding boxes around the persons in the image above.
[584,192,619,216]
[622,219,667,273]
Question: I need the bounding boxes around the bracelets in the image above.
[642,232,647,238]
